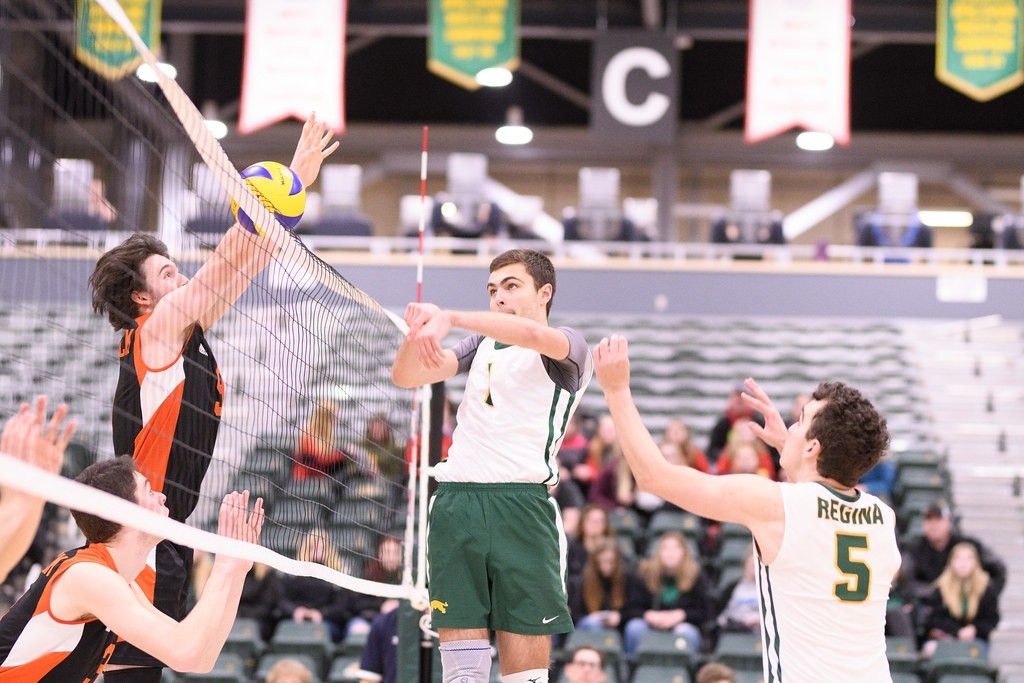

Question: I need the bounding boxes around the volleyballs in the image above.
[229,161,307,238]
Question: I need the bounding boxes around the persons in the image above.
[390,248,594,683]
[0,394,78,584]
[593,333,901,683]
[0,399,1008,683]
[0,454,264,683]
[88,111,340,682]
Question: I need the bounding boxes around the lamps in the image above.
[492,106,533,145]
[201,99,228,140]
[135,42,177,85]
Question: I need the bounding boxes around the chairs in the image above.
[158,433,763,683]
[923,640,998,683]
[907,515,959,537]
[310,161,371,236]
[896,489,950,519]
[564,168,633,239]
[863,172,922,245]
[713,168,782,243]
[433,152,504,238]
[886,637,921,683]
[39,157,101,231]
[188,161,234,230]
[895,465,943,500]
[894,451,940,471]
